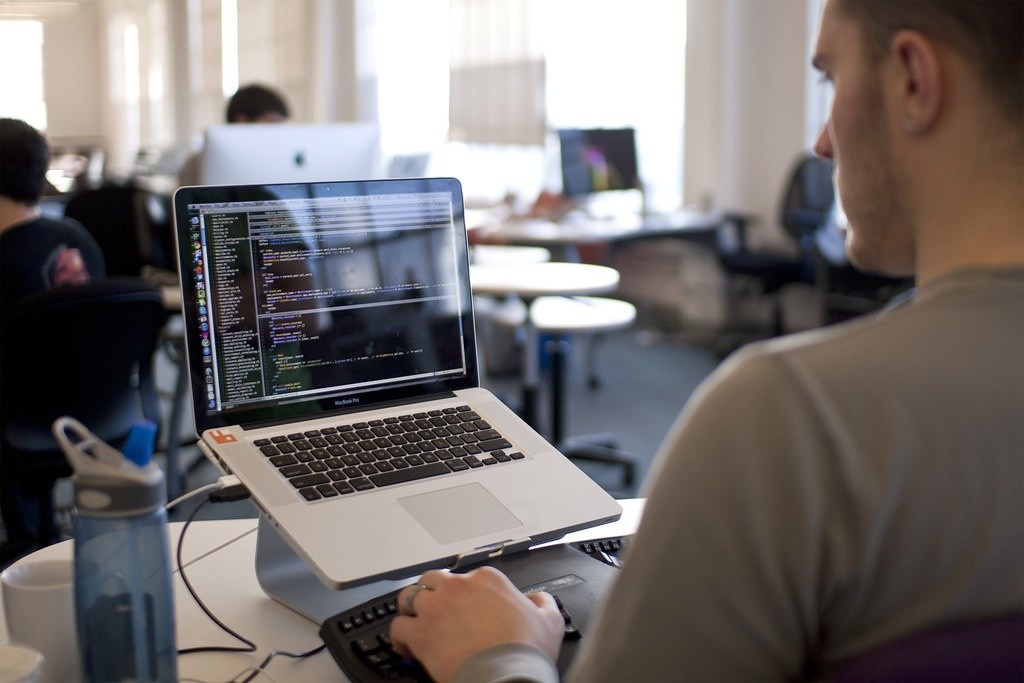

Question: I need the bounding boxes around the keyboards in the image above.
[319,536,634,683]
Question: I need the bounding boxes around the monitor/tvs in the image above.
[556,128,645,218]
[199,122,379,187]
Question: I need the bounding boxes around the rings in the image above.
[403,582,433,615]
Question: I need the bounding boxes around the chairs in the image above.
[0,289,170,550]
[710,156,917,344]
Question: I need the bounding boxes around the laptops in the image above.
[172,175,622,592]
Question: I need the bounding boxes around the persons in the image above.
[387,0,1024,683]
[178,80,291,188]
[780,146,904,328]
[0,115,109,575]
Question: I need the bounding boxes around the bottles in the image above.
[51,415,182,683]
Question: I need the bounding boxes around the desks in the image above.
[0,496,646,683]
[468,234,639,493]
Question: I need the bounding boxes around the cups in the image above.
[0,645,45,683]
[0,558,128,683]
